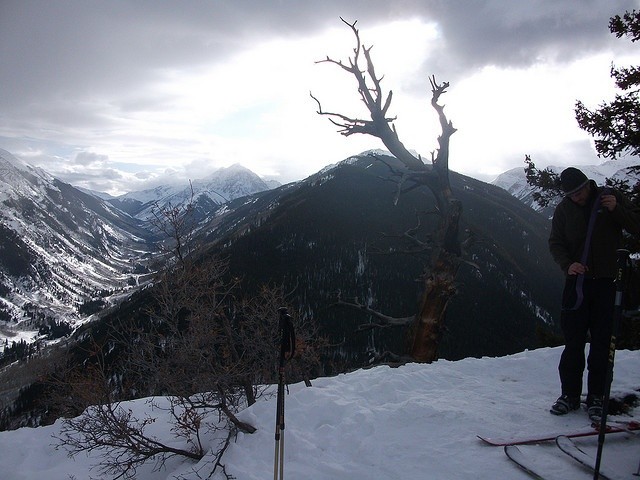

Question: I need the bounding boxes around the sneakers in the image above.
[586,395,604,421]
[550,394,580,415]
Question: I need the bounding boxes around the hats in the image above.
[561,167,589,197]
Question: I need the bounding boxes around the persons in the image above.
[548,167,640,421]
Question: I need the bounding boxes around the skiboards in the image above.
[476,421,639,446]
[503,433,625,479]
